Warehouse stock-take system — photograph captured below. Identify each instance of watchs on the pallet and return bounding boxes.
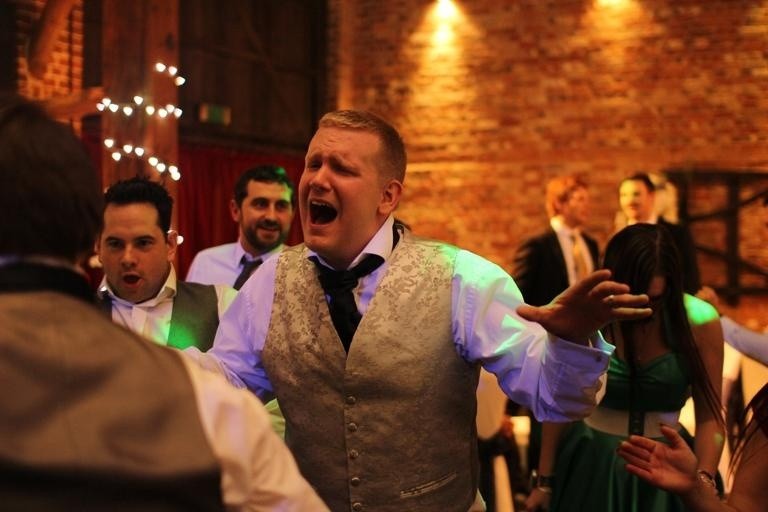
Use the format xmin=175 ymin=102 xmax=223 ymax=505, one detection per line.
xmin=528 ymin=471 xmax=554 ymax=491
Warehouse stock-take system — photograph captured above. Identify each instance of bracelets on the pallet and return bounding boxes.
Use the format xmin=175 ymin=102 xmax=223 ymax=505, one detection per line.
xmin=539 ymin=488 xmax=550 ymax=494
xmin=698 ymin=471 xmax=716 ymax=487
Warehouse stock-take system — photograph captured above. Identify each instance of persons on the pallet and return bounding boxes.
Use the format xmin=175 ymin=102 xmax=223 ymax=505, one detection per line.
xmin=1 ymin=95 xmax=324 ymax=512
xmin=617 ymin=384 xmax=768 ymax=511
xmin=206 ymin=110 xmax=652 ymax=512
xmin=619 ymin=173 xmax=701 ymax=292
xmin=527 ymin=223 xmax=725 ymax=507
xmin=186 ymin=163 xmax=292 ymax=315
xmin=513 ymin=174 xmax=602 ymax=306
xmin=88 ymin=177 xmax=238 ymax=352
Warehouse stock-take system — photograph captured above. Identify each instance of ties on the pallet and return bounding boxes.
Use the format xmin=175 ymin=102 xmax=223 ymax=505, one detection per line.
xmin=231 ymin=254 xmax=264 ymax=291
xmin=302 ymin=220 xmax=400 ymax=358
xmin=569 ymin=233 xmax=591 ymax=283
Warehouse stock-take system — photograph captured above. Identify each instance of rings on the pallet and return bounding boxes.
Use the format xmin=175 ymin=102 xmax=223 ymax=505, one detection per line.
xmin=609 ymin=295 xmax=616 ymax=308
